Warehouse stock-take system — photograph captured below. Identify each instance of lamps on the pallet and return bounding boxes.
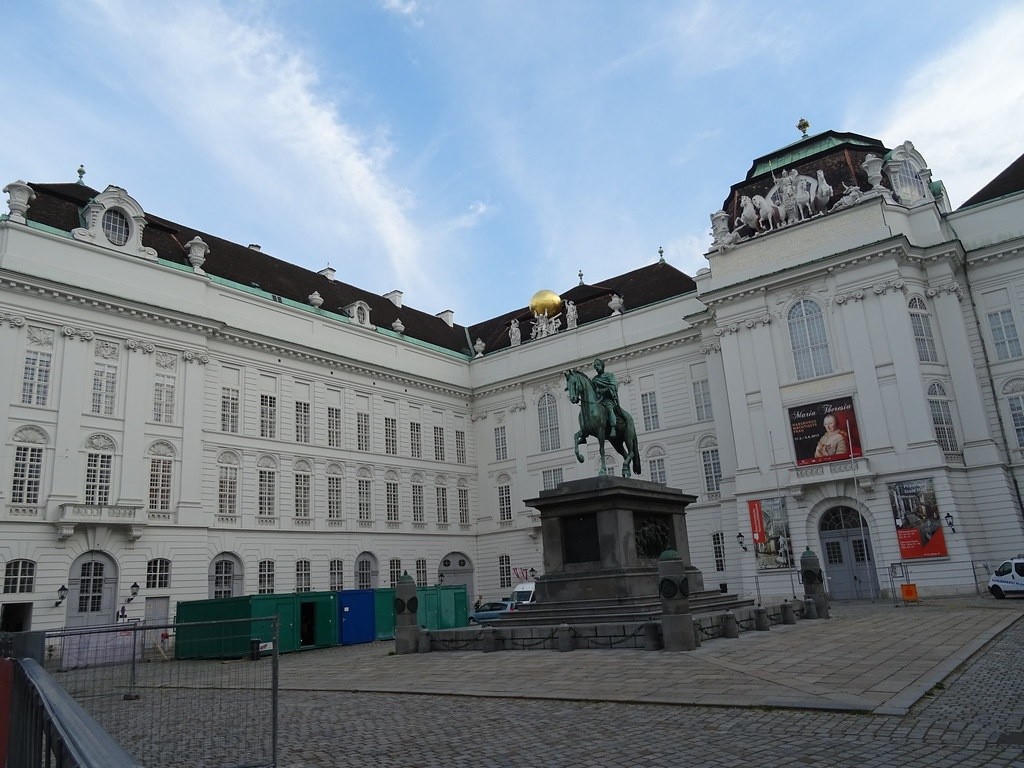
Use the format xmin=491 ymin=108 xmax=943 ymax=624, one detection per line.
xmin=736 ymin=532 xmax=748 ymax=551
xmin=528 ymin=567 xmax=537 ymax=578
xmin=434 ymin=573 xmax=445 ymax=587
xmin=55 ymin=584 xmax=68 ymax=606
xmin=945 ymin=513 xmax=956 ymax=533
xmin=127 ymin=582 xmax=140 ymax=604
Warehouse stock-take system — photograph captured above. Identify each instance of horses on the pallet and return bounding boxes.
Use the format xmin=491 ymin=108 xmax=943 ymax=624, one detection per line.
xmin=564 ymin=368 xmax=645 ymax=479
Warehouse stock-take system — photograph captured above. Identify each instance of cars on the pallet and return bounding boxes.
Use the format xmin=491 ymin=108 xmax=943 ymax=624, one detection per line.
xmin=469 ymin=601 xmax=522 ymax=624
xmin=987 ymin=558 xmax=1024 ymax=599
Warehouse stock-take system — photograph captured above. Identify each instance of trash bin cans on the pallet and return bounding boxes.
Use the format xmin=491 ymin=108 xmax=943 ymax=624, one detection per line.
xmin=900 ymin=583 xmax=918 ymax=600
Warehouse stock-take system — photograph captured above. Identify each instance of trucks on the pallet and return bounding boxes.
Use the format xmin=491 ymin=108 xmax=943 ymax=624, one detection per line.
xmin=508 ymin=582 xmax=536 ymax=604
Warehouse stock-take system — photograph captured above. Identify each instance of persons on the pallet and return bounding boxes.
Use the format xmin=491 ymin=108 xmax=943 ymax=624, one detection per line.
xmin=813 ymin=414 xmax=850 ymax=458
xmin=508 ymin=318 xmax=520 ymax=341
xmin=772 ymin=168 xmax=798 ymax=195
xmin=564 ymin=299 xmax=578 ymax=327
xmin=589 ymin=358 xmax=620 ymax=437
xmin=529 ymin=308 xmax=562 ymax=338
